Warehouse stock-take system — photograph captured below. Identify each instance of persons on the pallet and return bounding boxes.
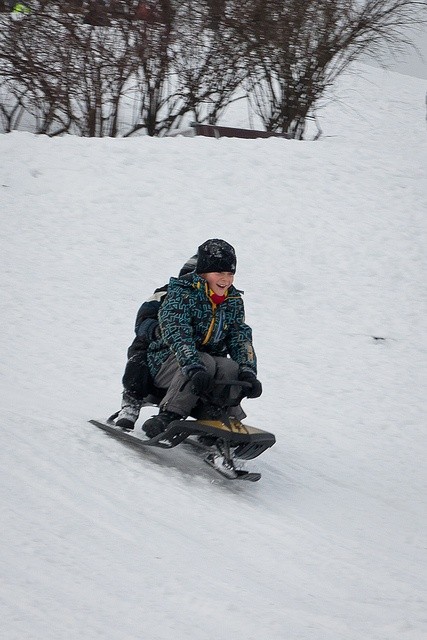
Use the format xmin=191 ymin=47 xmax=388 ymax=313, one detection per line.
xmin=114 ymin=254 xmax=199 ymax=429
xmin=142 ymin=239 xmax=263 ymax=438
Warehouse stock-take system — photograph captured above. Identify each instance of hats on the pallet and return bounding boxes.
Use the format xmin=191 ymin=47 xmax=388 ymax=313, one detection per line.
xmin=196 ymin=239 xmax=236 ymax=274
xmin=180 ymin=254 xmax=199 ymax=276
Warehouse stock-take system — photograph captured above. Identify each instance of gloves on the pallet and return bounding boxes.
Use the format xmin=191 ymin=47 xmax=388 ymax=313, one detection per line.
xmin=239 ymin=371 xmax=262 ymax=398
xmin=187 ymin=370 xmax=210 ymax=394
xmin=155 ymin=325 xmax=162 ymax=339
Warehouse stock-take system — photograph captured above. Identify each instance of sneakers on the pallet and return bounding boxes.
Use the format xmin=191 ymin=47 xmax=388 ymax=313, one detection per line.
xmin=142 ymin=412 xmax=181 ymax=439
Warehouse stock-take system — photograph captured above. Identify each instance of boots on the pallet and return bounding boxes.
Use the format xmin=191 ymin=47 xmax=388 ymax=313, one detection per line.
xmin=115 ymin=392 xmax=142 ymax=428
